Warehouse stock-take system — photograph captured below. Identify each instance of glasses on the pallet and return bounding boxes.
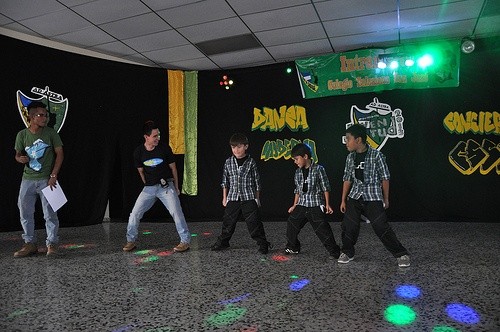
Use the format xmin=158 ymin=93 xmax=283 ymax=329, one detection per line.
xmin=33 ymin=114 xmax=50 ymax=118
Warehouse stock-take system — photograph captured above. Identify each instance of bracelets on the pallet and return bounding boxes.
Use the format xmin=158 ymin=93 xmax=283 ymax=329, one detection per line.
xmin=50 ymin=174 xmax=56 ymax=178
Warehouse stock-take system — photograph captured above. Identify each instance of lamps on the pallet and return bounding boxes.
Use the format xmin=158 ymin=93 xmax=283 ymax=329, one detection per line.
xmin=460 ymin=37 xmax=476 ymax=54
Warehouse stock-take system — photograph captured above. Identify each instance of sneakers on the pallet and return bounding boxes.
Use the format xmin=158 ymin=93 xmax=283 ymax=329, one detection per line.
xmin=258 ymin=244 xmax=267 ymax=254
xmin=286 ymin=246 xmax=301 ymax=254
xmin=14 ymin=244 xmax=36 ymax=258
xmin=336 ymin=253 xmax=354 ymax=263
xmin=46 ymin=245 xmax=56 ymax=258
xmin=175 ymin=241 xmax=190 ymax=253
xmin=123 ymin=241 xmax=137 ymax=251
xmin=397 ymin=253 xmax=412 ymax=267
xmin=329 ymin=250 xmax=339 ymax=260
xmin=210 ymin=242 xmax=231 ymax=250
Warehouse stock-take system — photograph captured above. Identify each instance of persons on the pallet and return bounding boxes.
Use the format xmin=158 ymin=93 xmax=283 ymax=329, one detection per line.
xmin=122 ymin=120 xmax=192 ymax=252
xmin=210 ymin=132 xmax=270 ymax=254
xmin=284 ymin=143 xmax=341 ymax=259
xmin=13 ymin=102 xmax=64 ymax=260
xmin=337 ymin=123 xmax=411 ymax=267
